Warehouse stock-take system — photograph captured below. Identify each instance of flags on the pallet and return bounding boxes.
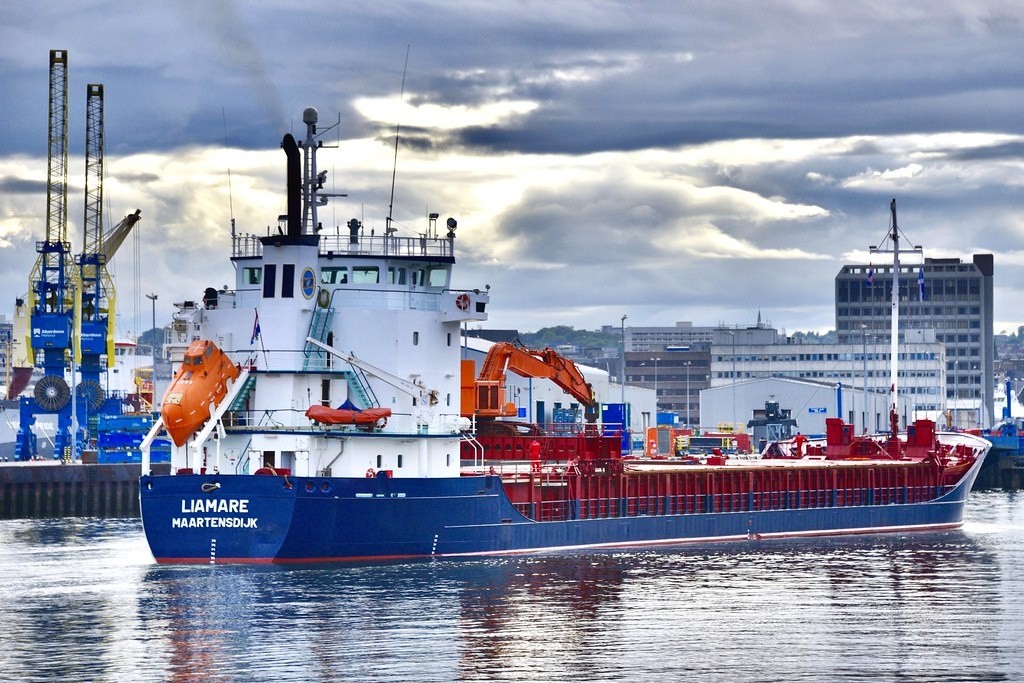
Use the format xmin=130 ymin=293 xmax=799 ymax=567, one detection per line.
xmin=918 ymin=262 xmax=925 ymax=303
xmin=867 ymin=261 xmax=873 ymax=285
xmin=251 ymin=313 xmax=261 ymax=345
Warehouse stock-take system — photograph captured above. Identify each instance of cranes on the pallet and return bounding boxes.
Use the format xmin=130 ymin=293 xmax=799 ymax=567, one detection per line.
xmin=76 ymin=83 xmax=142 ymax=463
xmin=8 ymin=50 xmax=73 ymax=465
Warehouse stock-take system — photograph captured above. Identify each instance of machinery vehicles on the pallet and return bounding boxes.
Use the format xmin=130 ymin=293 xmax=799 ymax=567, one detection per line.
xmin=460 ymin=337 xmax=601 ymax=450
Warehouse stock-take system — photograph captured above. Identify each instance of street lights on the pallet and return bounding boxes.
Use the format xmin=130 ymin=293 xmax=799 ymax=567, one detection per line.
xmin=727 ymin=330 xmax=737 ymax=423
xmin=144 ymin=292 xmax=158 ymax=385
xmin=684 ymin=360 xmax=693 ymax=428
xmin=620 ymin=314 xmax=628 ymax=403
xmin=649 ymin=358 xmax=661 ymax=393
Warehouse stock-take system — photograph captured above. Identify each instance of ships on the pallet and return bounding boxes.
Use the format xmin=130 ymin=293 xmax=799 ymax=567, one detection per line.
xmin=139 ymin=108 xmax=993 ymax=572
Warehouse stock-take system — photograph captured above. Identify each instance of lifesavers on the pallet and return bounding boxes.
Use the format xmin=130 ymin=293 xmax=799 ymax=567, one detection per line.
xmin=366 ymin=468 xmax=376 ymax=478
xmin=456 ymin=295 xmax=469 ymax=310
xmin=319 ymin=290 xmax=330 ymax=308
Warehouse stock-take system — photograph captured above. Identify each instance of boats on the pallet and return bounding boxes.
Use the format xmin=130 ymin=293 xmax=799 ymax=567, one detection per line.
xmin=160 ymin=338 xmax=242 ymax=448
xmin=303 ymin=404 xmax=393 ymax=428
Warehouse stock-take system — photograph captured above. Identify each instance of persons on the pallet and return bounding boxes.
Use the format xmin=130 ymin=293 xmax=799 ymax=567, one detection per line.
xmin=340 ymin=274 xmax=348 ymax=283
xmin=529 ymin=438 xmax=542 ymax=473
xmin=791 ymin=432 xmax=809 ymax=458
